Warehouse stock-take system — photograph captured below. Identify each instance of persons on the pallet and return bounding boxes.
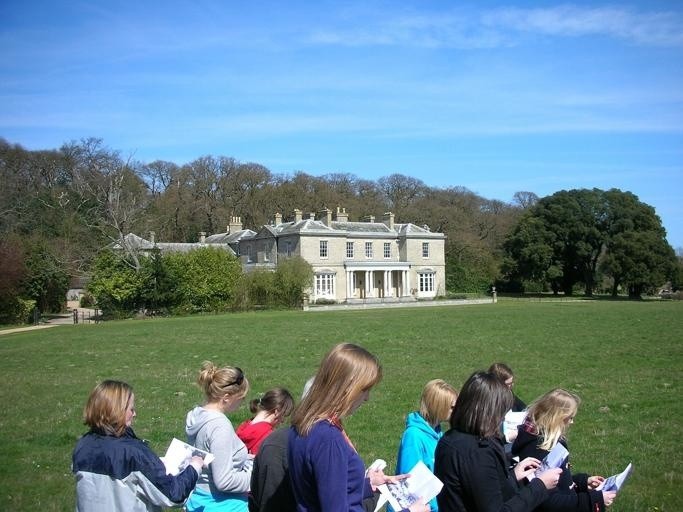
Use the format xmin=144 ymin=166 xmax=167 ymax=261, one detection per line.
xmin=247 ymin=374 xmax=323 ymax=511
xmin=69 ymin=379 xmax=203 ymax=511
xmin=511 ymin=386 xmax=617 ymax=511
xmin=486 ymin=364 xmax=525 ymax=443
xmin=384 ymin=376 xmax=456 ymax=511
xmin=178 ymin=356 xmax=261 ymax=512
xmin=431 ymin=370 xmax=564 ymax=511
xmin=285 ymin=341 xmax=433 ymax=512
xmin=238 ymin=387 xmax=295 ymax=457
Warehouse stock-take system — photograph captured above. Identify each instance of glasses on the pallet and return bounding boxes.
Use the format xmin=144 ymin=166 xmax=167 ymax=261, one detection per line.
xmin=220 ymin=366 xmax=245 ymax=388
